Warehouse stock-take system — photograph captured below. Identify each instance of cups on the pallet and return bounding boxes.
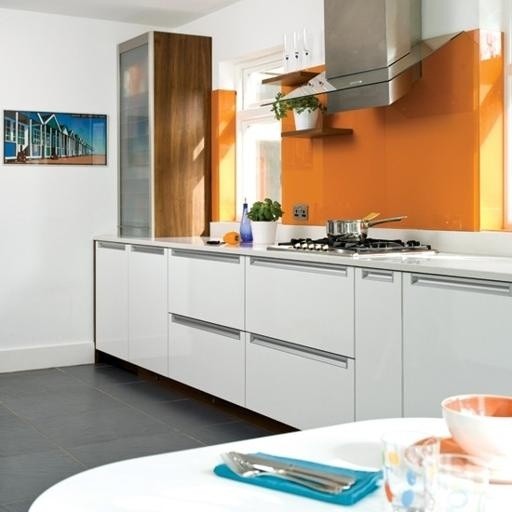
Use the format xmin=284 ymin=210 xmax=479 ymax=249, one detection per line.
xmin=383 ymin=451 xmax=432 ymax=512
xmin=426 ymin=455 xmax=486 ymax=511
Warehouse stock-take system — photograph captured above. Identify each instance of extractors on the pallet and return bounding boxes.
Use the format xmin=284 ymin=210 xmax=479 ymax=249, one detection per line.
xmin=261 ymin=0 xmax=464 ymax=114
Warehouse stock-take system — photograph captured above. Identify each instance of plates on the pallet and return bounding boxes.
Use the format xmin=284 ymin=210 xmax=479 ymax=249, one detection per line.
xmin=404 ymin=431 xmax=512 ymax=483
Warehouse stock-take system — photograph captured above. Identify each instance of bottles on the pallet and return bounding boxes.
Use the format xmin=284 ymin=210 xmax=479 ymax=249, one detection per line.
xmin=223 ymin=232 xmax=239 ymax=245
xmin=280 ymin=28 xmax=315 ymax=73
xmin=239 ymin=199 xmax=252 ymax=243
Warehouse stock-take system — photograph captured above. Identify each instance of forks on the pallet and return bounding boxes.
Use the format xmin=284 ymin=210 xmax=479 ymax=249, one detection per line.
xmin=219 ymin=452 xmax=341 ymax=495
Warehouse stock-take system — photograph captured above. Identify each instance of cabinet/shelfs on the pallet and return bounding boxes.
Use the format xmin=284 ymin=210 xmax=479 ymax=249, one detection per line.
xmin=245 ymin=255 xmax=355 ymax=433
xmin=262 ymin=71 xmax=353 ymax=139
xmin=94 ymin=240 xmax=167 ymax=379
xmin=165 ymin=247 xmax=245 ymax=412
xmin=115 ymin=30 xmax=211 ymax=238
xmin=401 ymin=271 xmax=512 ymax=418
xmin=355 ymin=266 xmax=401 ymax=423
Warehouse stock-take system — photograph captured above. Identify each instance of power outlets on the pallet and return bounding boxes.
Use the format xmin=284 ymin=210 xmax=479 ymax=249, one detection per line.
xmin=292 ymin=206 xmax=308 ymax=220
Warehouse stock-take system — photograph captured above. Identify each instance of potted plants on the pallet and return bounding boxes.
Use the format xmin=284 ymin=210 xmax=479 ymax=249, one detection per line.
xmin=271 ymin=93 xmax=327 ymax=132
xmin=246 ymin=198 xmax=285 ymax=251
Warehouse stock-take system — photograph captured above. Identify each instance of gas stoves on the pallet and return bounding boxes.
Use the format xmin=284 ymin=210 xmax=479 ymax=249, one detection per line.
xmin=266 ymin=236 xmax=441 ymax=258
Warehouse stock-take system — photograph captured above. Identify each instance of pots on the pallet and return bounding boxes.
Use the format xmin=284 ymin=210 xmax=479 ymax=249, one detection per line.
xmin=326 ymin=212 xmax=409 ymax=241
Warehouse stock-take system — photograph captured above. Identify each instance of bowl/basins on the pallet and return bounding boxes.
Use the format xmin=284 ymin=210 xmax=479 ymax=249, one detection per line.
xmin=441 ymin=394 xmax=512 ymax=467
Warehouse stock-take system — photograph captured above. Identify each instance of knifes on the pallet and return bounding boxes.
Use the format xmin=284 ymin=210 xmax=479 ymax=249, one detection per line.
xmin=249 ymin=462 xmax=350 ymax=490
xmin=232 ymin=452 xmax=355 ymax=486
